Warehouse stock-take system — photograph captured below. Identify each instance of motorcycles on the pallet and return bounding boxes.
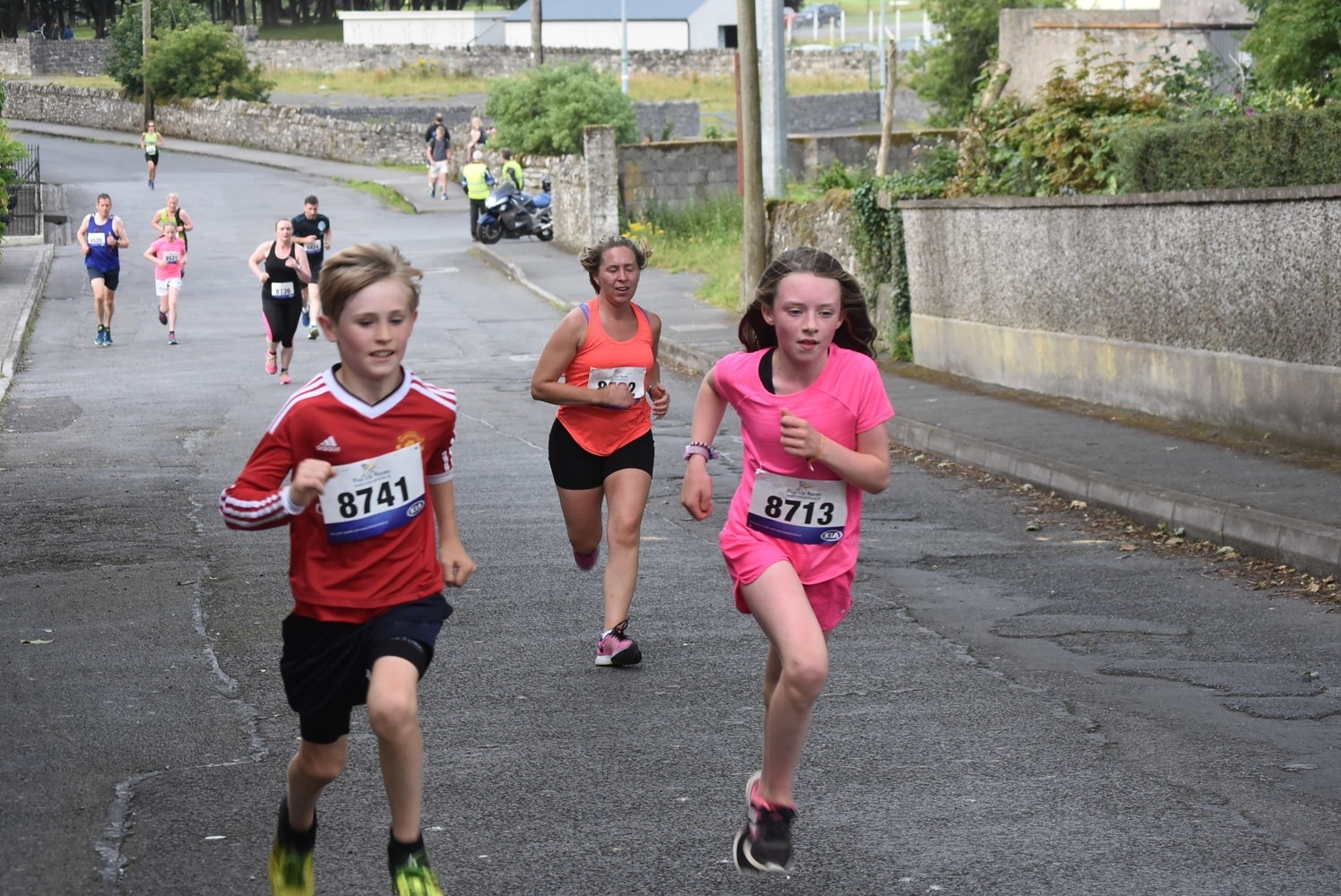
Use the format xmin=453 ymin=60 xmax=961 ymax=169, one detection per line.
xmin=475 ymin=176 xmax=553 ymax=244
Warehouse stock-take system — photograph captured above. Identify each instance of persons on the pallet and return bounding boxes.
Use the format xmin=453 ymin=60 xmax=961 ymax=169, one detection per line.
xmin=680 ymin=246 xmax=895 ymax=880
xmin=151 ymin=193 xmax=194 ymax=278
xmin=530 ymin=236 xmax=671 ymax=666
xmin=218 ymin=244 xmax=477 ymax=896
xmin=423 ymin=110 xmax=524 ymax=243
xmin=77 ymin=193 xmax=129 ymax=347
xmin=143 ymin=221 xmax=187 ymax=346
xmin=247 ymin=217 xmax=312 ymax=385
xmin=140 ymin=120 xmax=165 ymax=190
xmin=290 ymin=194 xmax=332 ymax=340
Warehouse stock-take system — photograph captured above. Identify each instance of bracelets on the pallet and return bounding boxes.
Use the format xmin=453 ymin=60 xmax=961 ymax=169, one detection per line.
xmin=296 ymin=266 xmax=302 ymax=273
xmin=689 ymin=441 xmax=719 ymax=461
xmin=647 ymin=383 xmax=658 ymax=399
xmin=182 ymin=225 xmax=186 ymax=231
xmin=808 ymin=433 xmax=825 ymax=472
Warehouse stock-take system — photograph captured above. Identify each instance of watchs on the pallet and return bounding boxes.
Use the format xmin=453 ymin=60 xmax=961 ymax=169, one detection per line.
xmin=684 ymin=445 xmax=711 ymax=463
xmin=114 ymin=240 xmax=120 ymax=247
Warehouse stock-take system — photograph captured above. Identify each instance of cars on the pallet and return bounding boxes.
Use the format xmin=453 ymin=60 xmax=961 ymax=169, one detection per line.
xmin=784 ymin=4 xmax=841 ymax=28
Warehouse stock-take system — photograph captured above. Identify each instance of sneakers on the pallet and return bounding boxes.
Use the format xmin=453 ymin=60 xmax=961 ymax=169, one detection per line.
xmin=594 ymin=617 xmax=642 ymax=666
xmin=268 ymin=796 xmax=317 ymax=896
xmin=266 ymin=348 xmax=278 ymax=374
xmin=279 ymin=371 xmax=293 ymax=385
xmin=103 ymin=329 xmax=114 ymax=346
xmin=308 ymin=326 xmax=320 ymax=340
xmin=94 ymin=324 xmax=105 ymax=345
xmin=168 ymin=333 xmax=178 ymax=345
xmin=743 ymin=769 xmax=797 ymax=873
xmin=387 ymin=842 xmax=444 ymax=896
xmin=733 ymin=819 xmax=761 ymax=877
xmin=158 ymin=304 xmax=168 ymax=325
xmin=573 ymin=545 xmax=599 ymax=572
xmin=302 ymin=303 xmax=310 ymax=327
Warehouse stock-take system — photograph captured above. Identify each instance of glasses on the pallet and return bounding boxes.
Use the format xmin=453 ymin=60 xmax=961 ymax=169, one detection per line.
xmin=148 ymin=125 xmax=154 ymax=128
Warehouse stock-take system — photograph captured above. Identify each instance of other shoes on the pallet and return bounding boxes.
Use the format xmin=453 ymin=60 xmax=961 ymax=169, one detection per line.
xmin=472 ymin=236 xmax=478 ymax=242
xmin=430 ymin=189 xmax=436 ymax=199
xmin=148 ymin=179 xmax=154 ymax=189
xmin=441 ymin=193 xmax=448 ymax=201
